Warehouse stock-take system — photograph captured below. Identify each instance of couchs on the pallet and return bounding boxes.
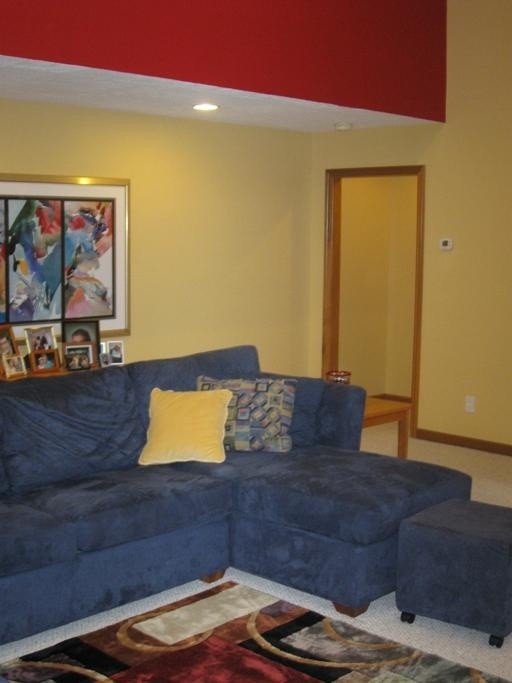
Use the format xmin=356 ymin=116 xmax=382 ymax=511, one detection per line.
xmin=0 ymin=344 xmax=512 ymax=647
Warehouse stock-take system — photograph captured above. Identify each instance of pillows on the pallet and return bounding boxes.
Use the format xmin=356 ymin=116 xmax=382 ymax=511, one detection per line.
xmin=194 ymin=373 xmax=297 ymax=454
xmin=138 ymin=386 xmax=234 ymax=466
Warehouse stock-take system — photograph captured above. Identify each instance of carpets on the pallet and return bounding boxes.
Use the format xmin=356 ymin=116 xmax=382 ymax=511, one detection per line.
xmin=1 ymin=580 xmax=512 ymax=682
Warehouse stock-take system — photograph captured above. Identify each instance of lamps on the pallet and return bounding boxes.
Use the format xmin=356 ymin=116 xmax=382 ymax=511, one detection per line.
xmin=193 ymin=102 xmax=220 ymax=112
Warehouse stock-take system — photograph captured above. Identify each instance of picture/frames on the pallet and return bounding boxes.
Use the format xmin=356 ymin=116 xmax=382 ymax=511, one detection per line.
xmin=1 ymin=352 xmax=26 ymax=378
xmin=23 ymin=323 xmax=59 ymax=377
xmin=65 ymin=344 xmax=93 ymax=370
xmin=61 ymin=320 xmax=104 ymax=373
xmin=0 ymin=324 xmax=22 ymax=380
xmin=67 ymin=354 xmax=90 ymax=371
xmin=0 ymin=170 xmax=132 ymax=339
xmin=29 ymin=347 xmax=62 ymax=375
xmin=105 ymin=340 xmax=124 ymax=365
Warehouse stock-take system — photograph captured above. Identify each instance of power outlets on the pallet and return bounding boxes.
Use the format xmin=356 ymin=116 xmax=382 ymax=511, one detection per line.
xmin=464 ymin=394 xmax=476 ymax=413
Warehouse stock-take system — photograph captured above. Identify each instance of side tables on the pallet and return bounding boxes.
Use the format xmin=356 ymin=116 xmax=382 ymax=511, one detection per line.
xmin=360 ymin=394 xmax=415 ymax=462
